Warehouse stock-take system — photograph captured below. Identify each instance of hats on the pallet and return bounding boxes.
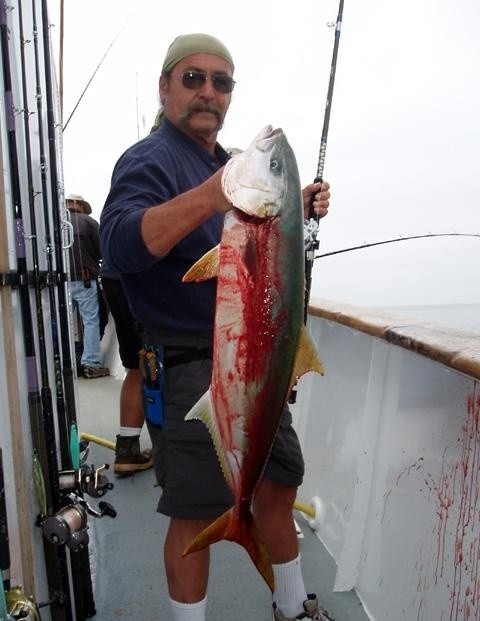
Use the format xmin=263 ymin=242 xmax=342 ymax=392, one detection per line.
xmin=65 ymin=193 xmax=92 ymax=214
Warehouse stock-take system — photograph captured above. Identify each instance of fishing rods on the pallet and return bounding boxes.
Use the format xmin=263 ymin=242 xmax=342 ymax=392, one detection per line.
xmin=314 ymin=234 xmax=478 ymax=259
xmin=1 ymin=0 xmax=97 ymax=620
xmin=63 ymin=26 xmax=140 ymax=142
xmin=288 ymin=0 xmax=343 ymax=403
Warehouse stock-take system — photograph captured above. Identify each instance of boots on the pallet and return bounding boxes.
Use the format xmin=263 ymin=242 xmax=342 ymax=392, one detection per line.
xmin=113 ymin=434 xmax=154 ymax=474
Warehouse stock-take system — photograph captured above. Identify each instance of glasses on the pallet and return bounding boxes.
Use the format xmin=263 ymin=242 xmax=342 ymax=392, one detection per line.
xmin=179 ymin=70 xmax=236 ymax=94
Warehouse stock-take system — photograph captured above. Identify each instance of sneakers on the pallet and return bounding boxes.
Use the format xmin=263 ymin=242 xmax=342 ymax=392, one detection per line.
xmin=272 ymin=594 xmax=339 ymax=621
xmin=82 ymin=363 xmax=109 ymax=379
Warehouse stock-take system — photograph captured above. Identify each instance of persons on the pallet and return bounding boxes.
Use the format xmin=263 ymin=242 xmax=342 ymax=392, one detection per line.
xmin=65 ymin=194 xmax=110 ymax=379
xmin=97 ymin=28 xmax=341 ymax=621
xmin=99 ymin=254 xmax=155 ymax=475
